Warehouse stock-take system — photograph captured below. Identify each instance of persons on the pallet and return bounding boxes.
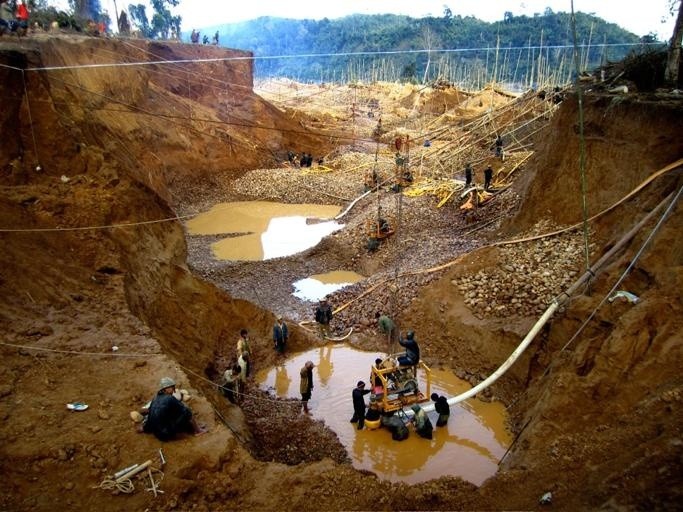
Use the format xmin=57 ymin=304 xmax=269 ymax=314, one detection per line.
xmin=484 ymin=165 xmax=493 ymax=189
xmin=0 ymin=0 xmax=30 ymax=38
xmin=288 ymin=151 xmax=312 ymax=167
xmin=315 ymin=298 xmax=333 ymax=338
xmin=465 ymin=163 xmax=473 ymax=186
xmin=135 ymin=377 xmax=209 ymax=441
xmin=221 ymin=329 xmax=252 ymax=403
xmin=398 ymin=330 xmax=419 ymax=366
xmin=350 ymin=358 xmax=450 ymax=440
xmin=496 ymin=136 xmax=502 ymax=157
xmin=375 ymin=312 xmax=398 ymax=344
xmin=300 ymin=360 xmax=314 ymax=414
xmin=273 ymin=315 xmax=289 ymax=358
xmin=191 ymin=28 xmax=220 ymax=45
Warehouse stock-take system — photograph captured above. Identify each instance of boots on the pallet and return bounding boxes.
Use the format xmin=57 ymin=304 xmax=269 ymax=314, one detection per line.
xmin=303 ymin=402 xmax=312 ymax=412
xmin=278 ymin=351 xmax=288 ymax=358
xmin=190 ymin=416 xmax=208 ymax=436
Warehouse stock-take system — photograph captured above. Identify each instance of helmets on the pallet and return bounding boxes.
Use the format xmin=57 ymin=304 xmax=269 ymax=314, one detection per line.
xmin=277 ymin=315 xmax=283 ymax=320
xmin=406 ymin=331 xmax=414 ymax=336
xmin=357 ymin=381 xmax=365 ymax=388
xmin=159 ymin=377 xmax=176 ymax=391
xmin=319 ymin=297 xmax=327 ymax=302
xmin=488 ymin=165 xmax=492 ymax=167
